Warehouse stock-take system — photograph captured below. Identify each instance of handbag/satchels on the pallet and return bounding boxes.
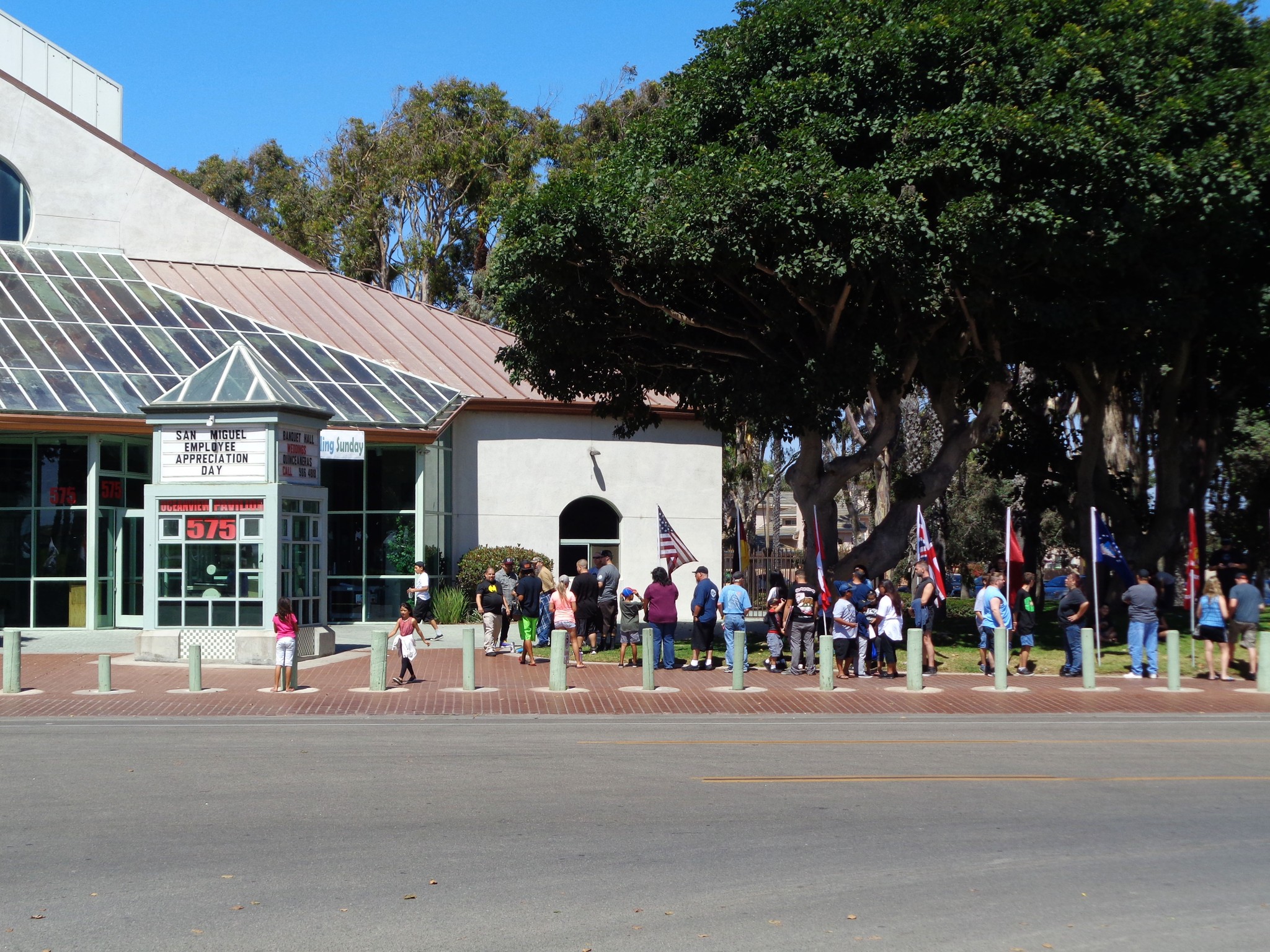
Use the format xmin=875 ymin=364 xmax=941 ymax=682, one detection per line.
xmin=512 ymin=596 xmax=522 ymax=620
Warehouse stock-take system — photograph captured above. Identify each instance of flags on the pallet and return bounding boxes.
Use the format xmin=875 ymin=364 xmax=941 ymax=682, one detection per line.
xmin=813 ymin=509 xmax=834 ymax=619
xmin=916 ymin=505 xmax=947 ymax=609
xmin=1006 ymin=507 xmax=1026 ymax=611
xmin=1090 ymin=507 xmax=1136 ymax=588
xmin=656 ymin=505 xmax=699 ymax=581
xmin=731 ymin=504 xmax=750 ymax=575
xmin=1182 ymin=511 xmax=1200 ymax=611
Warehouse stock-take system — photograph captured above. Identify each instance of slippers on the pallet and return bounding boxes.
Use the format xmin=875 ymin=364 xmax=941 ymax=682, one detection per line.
xmin=528 ymin=661 xmax=537 ymax=665
xmin=270 ymin=689 xmax=277 ymax=692
xmin=518 ymin=656 xmax=525 ymax=664
xmin=288 ymin=687 xmax=295 ymax=692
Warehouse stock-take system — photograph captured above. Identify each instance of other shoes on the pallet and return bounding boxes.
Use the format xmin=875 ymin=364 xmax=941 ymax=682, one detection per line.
xmin=393 ymin=678 xmax=402 ymax=685
xmin=570 ymin=640 xmax=639 ymax=668
xmin=534 ymin=642 xmax=549 ymax=649
xmin=407 ymin=676 xmax=416 ymax=682
xmin=486 ymin=652 xmax=496 ymax=656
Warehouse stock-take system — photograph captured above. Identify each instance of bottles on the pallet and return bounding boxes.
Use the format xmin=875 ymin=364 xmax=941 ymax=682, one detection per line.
xmin=516 ymin=648 xmax=523 ymax=653
xmin=408 ymin=588 xmax=413 ymax=599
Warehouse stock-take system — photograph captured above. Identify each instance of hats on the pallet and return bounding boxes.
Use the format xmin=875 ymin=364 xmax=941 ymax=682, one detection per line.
xmin=1235 ymin=573 xmax=1248 ymax=579
xmin=692 ymin=566 xmax=708 ymax=574
xmin=622 ymin=587 xmax=636 ymax=597
xmin=1139 ymin=569 xmax=1149 ymax=578
xmin=598 ymin=550 xmax=613 ymax=557
xmin=839 ymin=584 xmax=856 ymax=593
xmin=858 ymin=600 xmax=871 ymax=607
xmin=415 ymin=561 xmax=426 ymax=568
xmin=732 ymin=571 xmax=747 ymax=579
xmin=503 ymin=557 xmax=543 ymax=571
xmin=559 ymin=575 xmax=570 ymax=584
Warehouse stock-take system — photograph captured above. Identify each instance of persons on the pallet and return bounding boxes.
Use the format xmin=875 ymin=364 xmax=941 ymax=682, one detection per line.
xmin=643 ymin=567 xmax=679 ymax=670
xmin=388 ymin=602 xmax=430 ymax=685
xmin=681 ymin=566 xmax=719 ymax=671
xmin=476 ymin=557 xmax=558 ymax=666
xmin=270 ymin=599 xmax=299 ymax=692
xmin=909 ymin=560 xmax=937 ymax=676
xmin=1196 ymin=535 xmax=1265 ymax=682
xmin=973 ymin=556 xmax=1036 ymax=677
xmin=717 ymin=571 xmax=752 ymax=673
xmin=225 ymin=558 xmax=249 ymax=598
xmin=617 ymin=587 xmax=644 ymax=668
xmin=762 ymin=565 xmax=906 ymax=679
xmin=1043 ymin=549 xmax=1175 ymax=679
xmin=407 ymin=562 xmax=444 ymax=641
xmin=570 ymin=549 xmax=620 ymax=655
xmin=548 ymin=575 xmax=587 ymax=669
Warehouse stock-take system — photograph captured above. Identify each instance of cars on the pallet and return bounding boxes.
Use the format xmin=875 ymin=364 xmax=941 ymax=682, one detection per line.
xmin=754 ymin=573 xmax=792 ymax=592
xmin=944 ymin=573 xmax=984 ymax=598
xmin=1041 ymin=575 xmax=1087 ymax=602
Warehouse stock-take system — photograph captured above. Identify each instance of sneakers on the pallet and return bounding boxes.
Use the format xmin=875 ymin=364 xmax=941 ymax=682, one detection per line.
xmin=654 ymin=659 xmax=936 ymax=678
xmin=430 ymin=634 xmax=443 ymax=641
xmin=980 ymin=665 xmax=1255 ymax=681
xmin=499 ymin=641 xmax=511 ymax=647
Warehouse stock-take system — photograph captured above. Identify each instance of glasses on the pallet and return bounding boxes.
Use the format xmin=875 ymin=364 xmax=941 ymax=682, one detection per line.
xmin=867 ymin=598 xmax=876 ymax=602
xmin=593 ymin=556 xmax=600 ymax=559
xmin=879 ymin=586 xmax=884 ymax=588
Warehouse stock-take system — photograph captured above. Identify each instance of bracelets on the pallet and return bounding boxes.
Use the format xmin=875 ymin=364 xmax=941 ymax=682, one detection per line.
xmin=514 ymin=593 xmax=517 ymax=594
xmin=721 ymin=615 xmax=724 ymax=617
xmin=638 ymin=596 xmax=641 ymax=599
xmin=693 ymin=615 xmax=697 ymax=618
